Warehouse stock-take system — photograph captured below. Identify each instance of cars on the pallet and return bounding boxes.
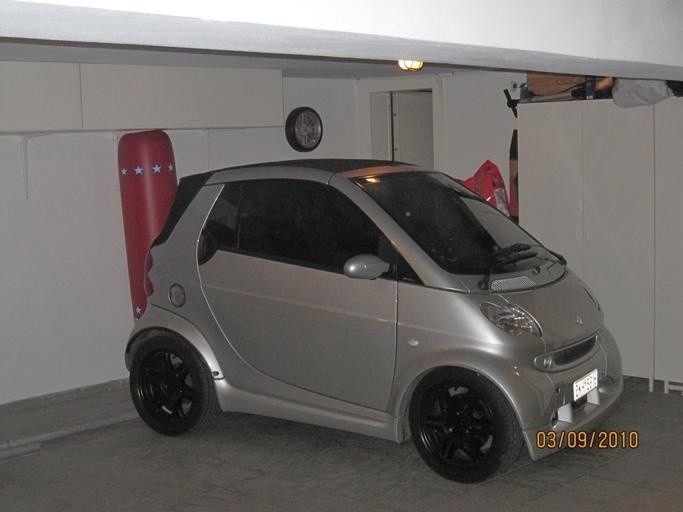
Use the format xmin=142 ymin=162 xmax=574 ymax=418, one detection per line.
xmin=124 ymin=158 xmax=624 ymax=484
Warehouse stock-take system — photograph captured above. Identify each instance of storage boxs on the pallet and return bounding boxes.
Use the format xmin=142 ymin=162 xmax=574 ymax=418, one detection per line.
xmin=613 ymin=79 xmax=672 ymax=108
xmin=527 ymin=77 xmax=590 ymax=93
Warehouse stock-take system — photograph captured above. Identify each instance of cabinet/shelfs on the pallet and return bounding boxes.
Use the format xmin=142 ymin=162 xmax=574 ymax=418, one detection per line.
xmin=520 ymin=100 xmax=655 ymax=391
xmin=653 ymin=100 xmax=682 ymax=393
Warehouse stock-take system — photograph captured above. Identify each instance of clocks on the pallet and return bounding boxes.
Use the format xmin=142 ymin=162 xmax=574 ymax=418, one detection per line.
xmin=285 ymin=107 xmax=324 ymax=152
